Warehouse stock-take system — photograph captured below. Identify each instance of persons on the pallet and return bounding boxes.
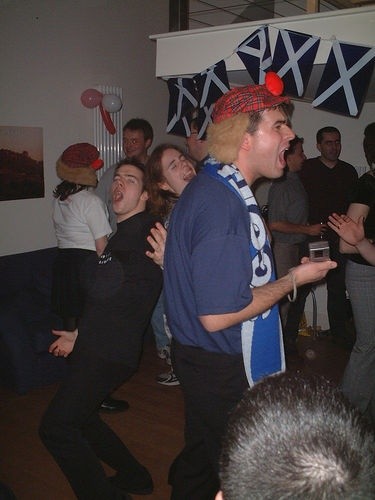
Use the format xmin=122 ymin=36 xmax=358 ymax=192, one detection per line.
xmin=38 ymin=155 xmax=163 ymax=500
xmin=163 ymin=71 xmax=338 ymax=500
xmin=267 ymin=122 xmax=375 ymax=425
xmin=51 ymin=106 xmax=211 ymax=410
xmin=216 ymin=370 xmax=375 ymax=500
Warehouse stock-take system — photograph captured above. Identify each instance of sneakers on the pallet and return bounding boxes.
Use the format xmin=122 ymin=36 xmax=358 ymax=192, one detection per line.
xmin=155 ymin=370 xmax=180 ymax=386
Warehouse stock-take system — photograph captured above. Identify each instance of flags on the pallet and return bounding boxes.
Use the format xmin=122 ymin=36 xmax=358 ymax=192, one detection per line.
xmin=166 ymin=59 xmax=231 ymax=140
xmin=272 ymin=28 xmax=320 ymax=99
xmin=233 ymin=25 xmax=271 ymax=86
xmin=312 ymin=40 xmax=375 ymax=119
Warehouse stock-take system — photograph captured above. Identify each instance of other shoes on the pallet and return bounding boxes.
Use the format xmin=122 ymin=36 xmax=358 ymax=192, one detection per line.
xmin=101 ymin=398 xmax=129 ymax=411
xmin=109 ymin=473 xmax=153 ymax=495
xmin=331 ymin=336 xmax=355 ymax=351
xmin=286 ymin=345 xmax=299 ymax=370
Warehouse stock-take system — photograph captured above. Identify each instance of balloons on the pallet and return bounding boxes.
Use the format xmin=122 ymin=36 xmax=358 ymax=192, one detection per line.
xmin=81 ymin=89 xmax=121 ymax=134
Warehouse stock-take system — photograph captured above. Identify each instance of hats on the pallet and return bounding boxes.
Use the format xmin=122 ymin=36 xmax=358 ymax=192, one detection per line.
xmin=56 ymin=142 xmax=103 ymax=187
xmin=210 ymin=71 xmax=291 ymax=123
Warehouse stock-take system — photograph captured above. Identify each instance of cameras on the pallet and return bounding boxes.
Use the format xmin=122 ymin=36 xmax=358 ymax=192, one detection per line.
xmin=308 ymin=241 xmax=331 ymax=262
